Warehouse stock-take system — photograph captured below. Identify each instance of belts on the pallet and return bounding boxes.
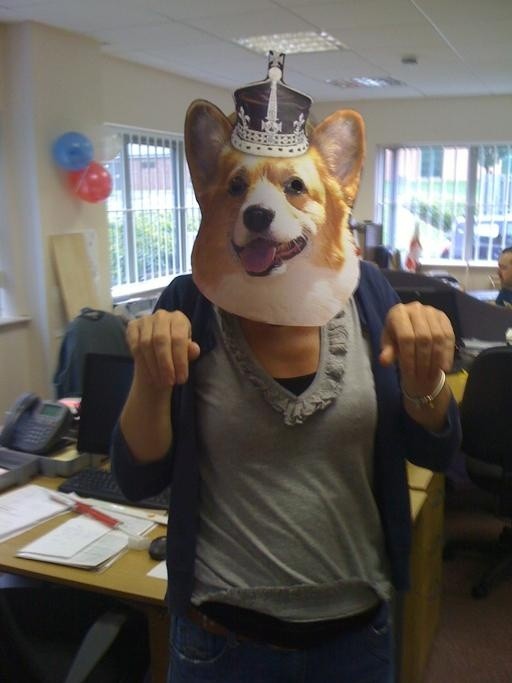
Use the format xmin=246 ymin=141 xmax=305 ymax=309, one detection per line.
xmin=181 ymin=602 xmax=297 ymax=653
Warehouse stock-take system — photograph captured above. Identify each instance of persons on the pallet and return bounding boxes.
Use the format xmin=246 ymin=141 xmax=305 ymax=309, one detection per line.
xmin=111 ymin=43 xmax=468 ymax=681
xmin=493 ymin=245 xmax=512 ymax=307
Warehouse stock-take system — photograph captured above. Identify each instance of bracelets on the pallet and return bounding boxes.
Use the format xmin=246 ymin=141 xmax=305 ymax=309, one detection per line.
xmin=399 ymin=369 xmax=446 ymax=411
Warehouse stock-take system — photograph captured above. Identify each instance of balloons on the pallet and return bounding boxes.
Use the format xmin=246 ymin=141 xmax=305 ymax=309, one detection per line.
xmin=69 ymin=160 xmax=114 ymax=202
xmin=50 ymin=127 xmax=96 ymax=171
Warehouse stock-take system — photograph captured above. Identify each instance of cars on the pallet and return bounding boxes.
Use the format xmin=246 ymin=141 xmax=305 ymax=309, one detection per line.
xmin=453 ymin=214 xmax=512 ymax=261
xmin=378 ymin=199 xmax=454 ymax=262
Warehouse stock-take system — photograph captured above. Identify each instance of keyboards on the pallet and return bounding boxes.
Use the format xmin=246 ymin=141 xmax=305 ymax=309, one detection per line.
xmin=58 ymin=466 xmax=168 ymax=510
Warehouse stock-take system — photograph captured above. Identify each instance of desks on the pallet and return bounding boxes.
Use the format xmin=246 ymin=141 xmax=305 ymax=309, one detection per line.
xmin=406 ymin=459 xmax=445 ymax=683
xmin=0 ymin=475 xmax=428 ymax=683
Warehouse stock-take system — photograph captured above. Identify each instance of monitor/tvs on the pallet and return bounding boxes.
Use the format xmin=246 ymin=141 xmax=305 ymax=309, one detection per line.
xmin=77 ymin=353 xmax=134 ymax=455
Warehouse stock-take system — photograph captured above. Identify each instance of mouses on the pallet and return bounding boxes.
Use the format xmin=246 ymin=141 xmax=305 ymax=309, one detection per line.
xmin=149 ymin=536 xmax=167 ymax=560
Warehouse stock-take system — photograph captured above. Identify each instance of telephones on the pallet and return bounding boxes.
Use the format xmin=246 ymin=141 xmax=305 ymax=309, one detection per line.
xmin=0 ymin=392 xmax=73 ymax=454
